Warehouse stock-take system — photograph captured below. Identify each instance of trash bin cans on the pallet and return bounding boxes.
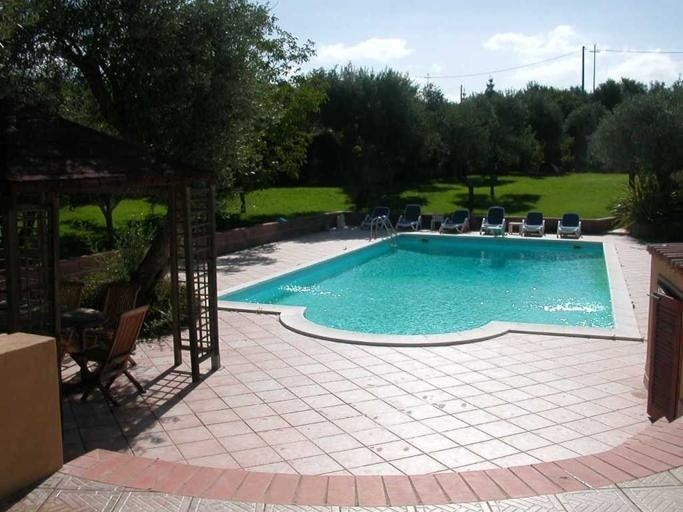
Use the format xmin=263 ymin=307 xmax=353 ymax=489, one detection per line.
xmin=337 ymin=214 xmax=344 ymax=228
xmin=644 ymin=242 xmax=683 ymax=417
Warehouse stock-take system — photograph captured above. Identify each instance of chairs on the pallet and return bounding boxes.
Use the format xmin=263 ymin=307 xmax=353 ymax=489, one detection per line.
xmin=359 ymin=204 xmax=582 ymax=240
xmin=50 ymin=283 xmax=151 ymax=451
